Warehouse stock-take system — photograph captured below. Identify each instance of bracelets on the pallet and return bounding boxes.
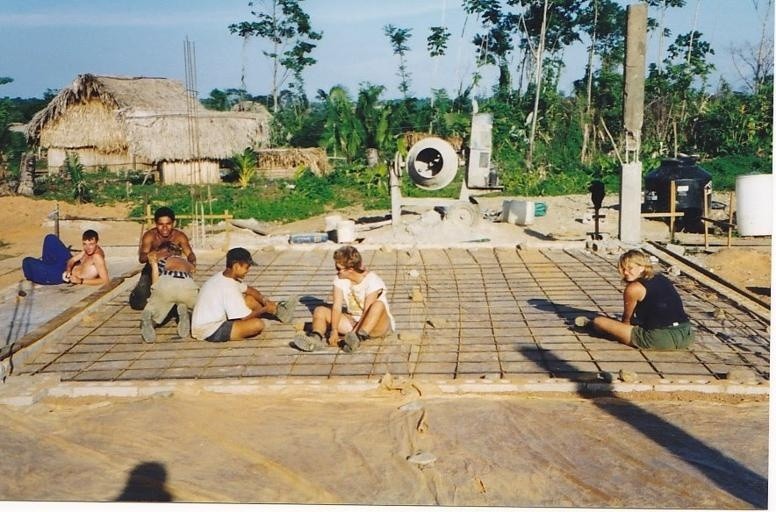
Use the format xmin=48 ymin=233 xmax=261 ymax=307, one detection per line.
xmin=80 ymin=278 xmax=84 ymax=284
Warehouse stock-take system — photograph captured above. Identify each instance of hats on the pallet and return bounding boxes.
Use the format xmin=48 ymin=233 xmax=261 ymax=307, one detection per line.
xmin=226 ymin=248 xmax=258 ymax=266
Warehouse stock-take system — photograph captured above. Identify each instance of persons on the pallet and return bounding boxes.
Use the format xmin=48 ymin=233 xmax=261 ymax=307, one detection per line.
xmin=572 ymin=250 xmax=693 ymax=351
xmin=293 ymin=245 xmax=394 ymax=352
xmin=141 ymin=242 xmax=196 ymax=343
xmin=189 ymin=247 xmax=295 ymax=343
xmin=128 ymin=206 xmax=196 ymax=310
xmin=22 ymin=229 xmax=108 ymax=285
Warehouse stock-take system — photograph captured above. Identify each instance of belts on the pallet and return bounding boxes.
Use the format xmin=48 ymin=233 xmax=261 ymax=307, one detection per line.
xmin=173 ymin=276 xmax=184 ymax=279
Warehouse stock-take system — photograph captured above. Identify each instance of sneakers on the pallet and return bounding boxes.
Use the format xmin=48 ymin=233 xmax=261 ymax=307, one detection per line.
xmin=176 ymin=303 xmax=191 ymax=337
xmin=141 ymin=309 xmax=156 ymax=343
xmin=343 ymin=332 xmax=360 ymax=352
xmin=293 ymin=333 xmax=325 ymax=351
xmin=335 ymin=266 xmax=347 ymax=271
xmin=574 ymin=316 xmax=591 ymax=328
xmin=275 ymin=294 xmax=297 ymax=324
